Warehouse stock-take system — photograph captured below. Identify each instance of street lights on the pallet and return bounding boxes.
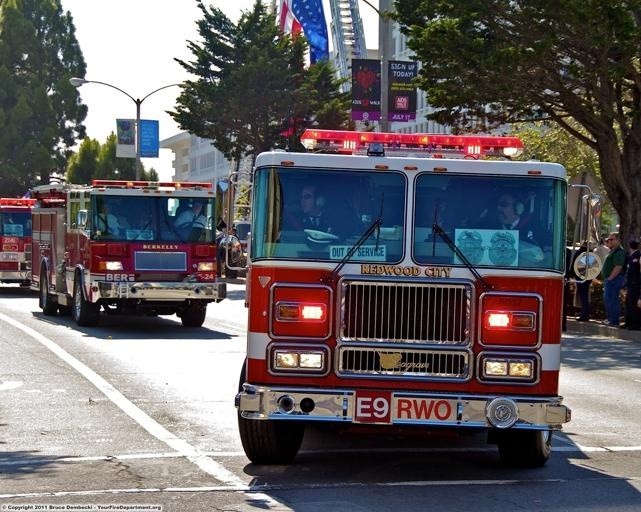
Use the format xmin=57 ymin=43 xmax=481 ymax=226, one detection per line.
xmin=69 ymin=77 xmax=188 ymax=181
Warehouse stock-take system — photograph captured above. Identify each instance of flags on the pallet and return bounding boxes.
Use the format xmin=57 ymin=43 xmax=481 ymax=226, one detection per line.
xmin=277 ymin=0 xmax=330 ymax=141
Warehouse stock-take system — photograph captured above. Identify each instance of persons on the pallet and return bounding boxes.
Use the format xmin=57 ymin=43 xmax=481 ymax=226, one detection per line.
xmin=574 ymin=239 xmax=595 ymax=323
xmin=620 ymin=237 xmax=641 ymax=331
xmin=593 ymin=237 xmax=612 ymax=259
xmin=175 ymin=198 xmax=207 ymax=229
xmin=95 ymin=203 xmax=121 ymax=237
xmin=288 ymin=180 xmax=342 ymax=238
xmin=215 ymin=220 xmax=241 ymax=279
xmin=494 ymin=193 xmax=540 ymax=248
xmin=601 ymin=233 xmax=626 ymax=326
xmin=113 ymin=204 xmax=131 ymax=228
xmin=562 ymin=247 xmax=573 ymax=333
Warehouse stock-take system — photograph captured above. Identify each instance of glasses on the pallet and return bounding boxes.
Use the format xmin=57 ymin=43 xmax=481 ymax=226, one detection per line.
xmin=609 ymin=238 xmax=613 ymax=240
xmin=495 ymin=200 xmax=516 ymax=207
xmin=300 ymin=194 xmax=317 ymax=200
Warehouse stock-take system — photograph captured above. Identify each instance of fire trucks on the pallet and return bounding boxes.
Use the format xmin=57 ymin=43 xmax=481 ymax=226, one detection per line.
xmin=234 ymin=128 xmax=601 ymax=466
xmin=30 ymin=179 xmax=227 ymax=329
xmin=0 ymin=197 xmax=36 ymax=285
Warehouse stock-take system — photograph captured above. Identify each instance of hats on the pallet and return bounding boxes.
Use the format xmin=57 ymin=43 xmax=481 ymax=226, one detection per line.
xmin=304 ymin=229 xmax=339 ymax=244
